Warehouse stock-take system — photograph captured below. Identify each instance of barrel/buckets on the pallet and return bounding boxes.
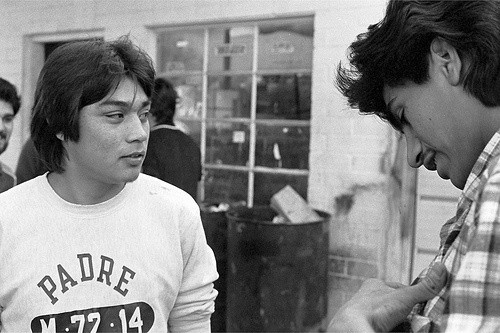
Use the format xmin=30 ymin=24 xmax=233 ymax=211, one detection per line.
xmin=226 ymin=205 xmax=332 ymax=332
xmin=198 ymin=201 xmax=248 ymax=332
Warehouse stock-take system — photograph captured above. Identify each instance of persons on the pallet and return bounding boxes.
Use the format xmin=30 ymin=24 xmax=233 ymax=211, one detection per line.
xmin=325 ymin=0 xmax=500 ymax=333
xmin=0 ymin=33 xmax=220 ymax=333
xmin=0 ymin=77 xmax=21 ymax=193
xmin=140 ymin=76 xmax=204 ymax=216
xmin=15 ymin=134 xmax=50 ymax=186
